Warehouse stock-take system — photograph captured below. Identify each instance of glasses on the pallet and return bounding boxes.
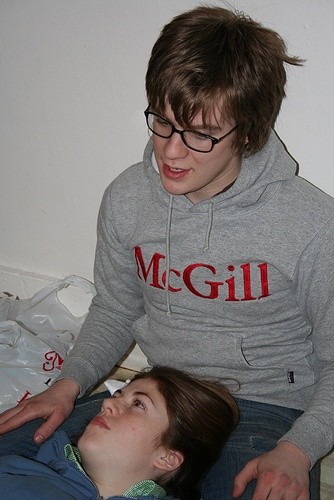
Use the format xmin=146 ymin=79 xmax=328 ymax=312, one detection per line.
xmin=144 ymin=103 xmax=240 ymax=153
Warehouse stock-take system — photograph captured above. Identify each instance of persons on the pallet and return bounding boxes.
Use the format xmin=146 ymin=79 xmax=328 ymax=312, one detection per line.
xmin=0 ymin=367 xmax=240 ymax=500
xmin=0 ymin=7 xmax=334 ymax=500
